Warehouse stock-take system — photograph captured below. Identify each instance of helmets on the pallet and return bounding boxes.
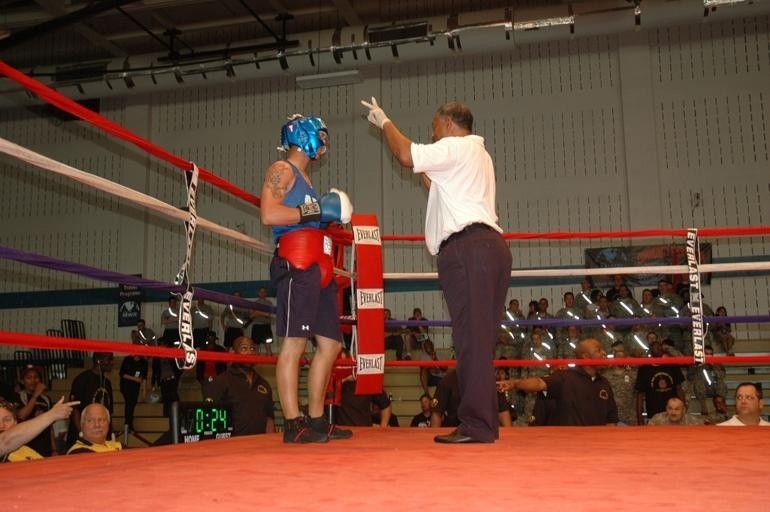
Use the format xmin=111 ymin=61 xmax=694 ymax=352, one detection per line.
xmin=277 ymin=114 xmax=328 ymax=160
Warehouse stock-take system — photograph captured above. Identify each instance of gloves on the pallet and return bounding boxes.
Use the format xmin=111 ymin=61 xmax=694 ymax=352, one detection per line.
xmin=319 ymin=188 xmax=353 ymax=224
xmin=361 ymin=97 xmax=391 ymax=130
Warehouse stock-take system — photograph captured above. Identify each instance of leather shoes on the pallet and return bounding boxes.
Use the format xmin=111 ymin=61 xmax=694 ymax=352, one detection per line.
xmin=434 ymin=430 xmax=477 ymax=442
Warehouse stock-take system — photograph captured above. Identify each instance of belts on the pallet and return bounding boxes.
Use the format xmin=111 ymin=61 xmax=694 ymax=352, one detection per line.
xmin=440 ymin=224 xmax=493 ymax=245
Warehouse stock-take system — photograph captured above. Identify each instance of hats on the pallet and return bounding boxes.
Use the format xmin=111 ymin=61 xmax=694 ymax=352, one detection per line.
xmin=20 ymin=364 xmax=44 ymax=379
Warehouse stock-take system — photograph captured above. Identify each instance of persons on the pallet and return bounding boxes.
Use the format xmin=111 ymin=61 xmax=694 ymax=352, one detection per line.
xmin=497 ymin=275 xmax=735 ymax=425
xmin=370 ymin=399 xmax=400 ymax=428
xmin=382 ymin=307 xmax=403 ymax=361
xmin=430 ymin=361 xmax=512 ymax=425
xmin=360 ymin=95 xmax=516 ymax=445
xmin=120 ymin=286 xmax=275 ymax=435
xmin=404 ymin=308 xmax=430 ymax=359
xmin=316 ymin=340 xmax=393 ymax=428
xmin=1 ymin=363 xmax=130 ymax=464
xmin=260 ymin=109 xmax=357 ymax=442
xmin=203 ymin=335 xmax=275 ymax=438
xmin=411 ymin=394 xmax=432 ymax=426
xmin=419 ymin=338 xmax=448 ymax=394
xmin=719 ymin=382 xmax=770 ymax=425
xmin=66 ymin=351 xmax=114 ymax=444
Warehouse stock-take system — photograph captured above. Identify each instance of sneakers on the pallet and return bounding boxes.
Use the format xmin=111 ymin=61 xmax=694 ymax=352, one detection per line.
xmin=283 ymin=415 xmax=352 ymax=443
